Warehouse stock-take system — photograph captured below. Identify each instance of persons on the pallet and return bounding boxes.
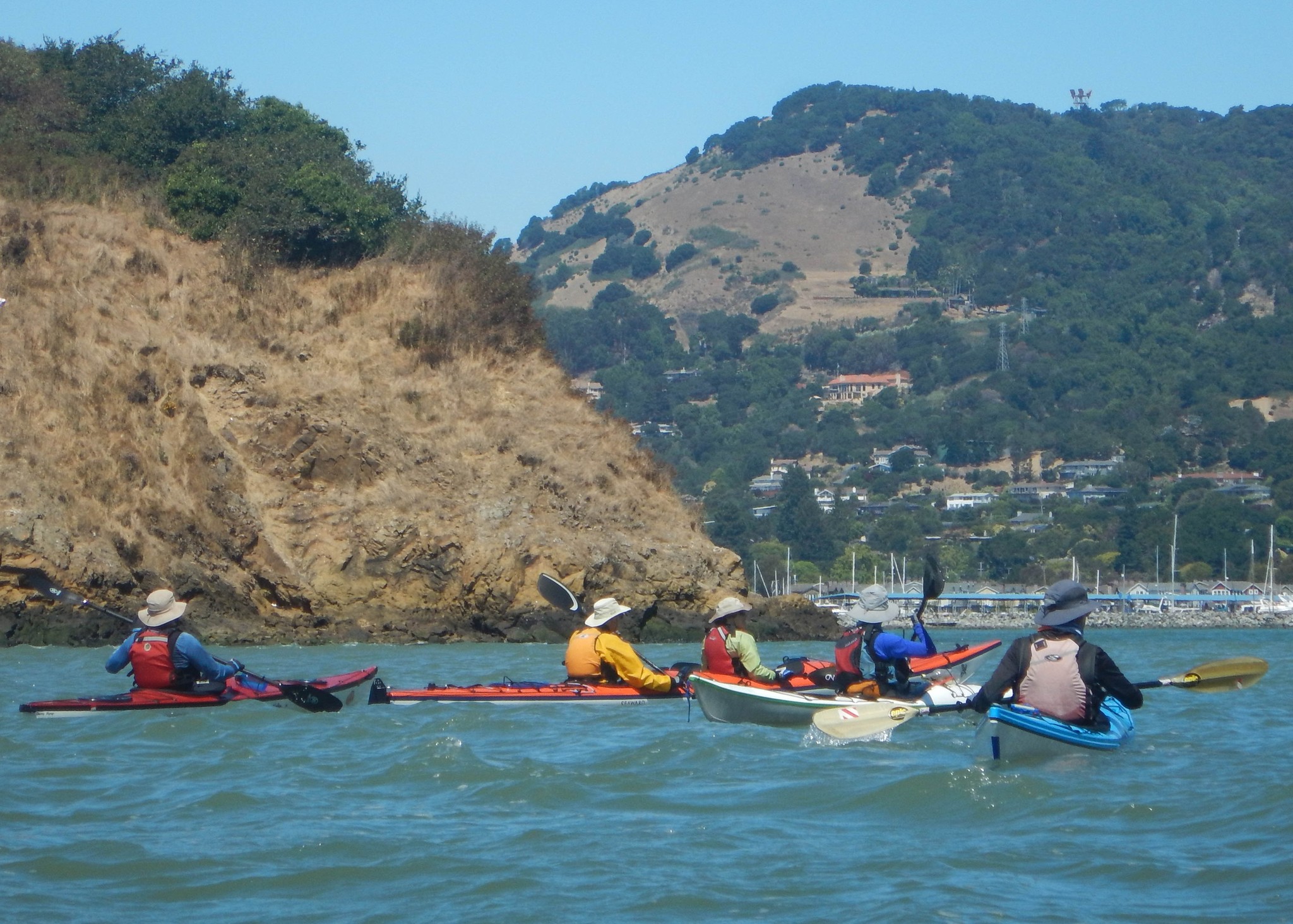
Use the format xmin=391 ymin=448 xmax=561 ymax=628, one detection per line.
xmin=700 ymin=595 xmax=795 ymax=684
xmin=846 ymin=584 xmax=938 ymax=700
xmin=564 ymin=598 xmax=683 ymax=694
xmin=966 ymin=579 xmax=1144 ymax=733
xmin=104 ymin=590 xmax=245 ymax=693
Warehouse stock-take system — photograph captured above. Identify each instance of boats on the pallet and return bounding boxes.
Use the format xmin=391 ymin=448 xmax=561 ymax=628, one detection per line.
xmin=968 ymin=694 xmax=1138 ymax=758
xmin=368 ymin=657 xmax=838 ymax=707
xmin=19 ymin=662 xmax=381 ymax=718
xmin=682 ymin=667 xmax=1019 ymax=730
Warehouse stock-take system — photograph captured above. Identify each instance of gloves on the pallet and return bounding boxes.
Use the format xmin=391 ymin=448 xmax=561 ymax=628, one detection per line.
xmin=911 ymin=613 xmax=924 ymax=624
xmin=779 ymin=669 xmax=794 ymax=683
xmin=674 ymin=666 xmax=693 ymax=685
xmin=227 ymin=659 xmax=246 ymax=672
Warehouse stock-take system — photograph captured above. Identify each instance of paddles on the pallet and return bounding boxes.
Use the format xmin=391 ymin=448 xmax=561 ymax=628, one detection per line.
xmin=911 ymin=554 xmax=945 ymax=642
xmin=813 ymin=657 xmax=1271 ymax=739
xmin=536 ymin=572 xmax=697 ymax=699
xmin=671 ymin=662 xmax=839 ymax=690
xmin=26 ymin=567 xmax=343 ymax=713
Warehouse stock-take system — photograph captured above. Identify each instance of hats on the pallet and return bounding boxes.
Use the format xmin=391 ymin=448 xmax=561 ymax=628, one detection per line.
xmin=138 ymin=589 xmax=187 ymax=627
xmin=846 ymin=584 xmax=899 ymax=623
xmin=709 ymin=598 xmax=752 ymax=624
xmin=585 ymin=598 xmax=631 ymax=627
xmin=1034 ymin=579 xmax=1098 ymax=625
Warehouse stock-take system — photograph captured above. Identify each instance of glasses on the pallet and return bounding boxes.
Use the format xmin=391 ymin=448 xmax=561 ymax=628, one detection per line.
xmin=736 ymin=611 xmax=747 ymax=616
